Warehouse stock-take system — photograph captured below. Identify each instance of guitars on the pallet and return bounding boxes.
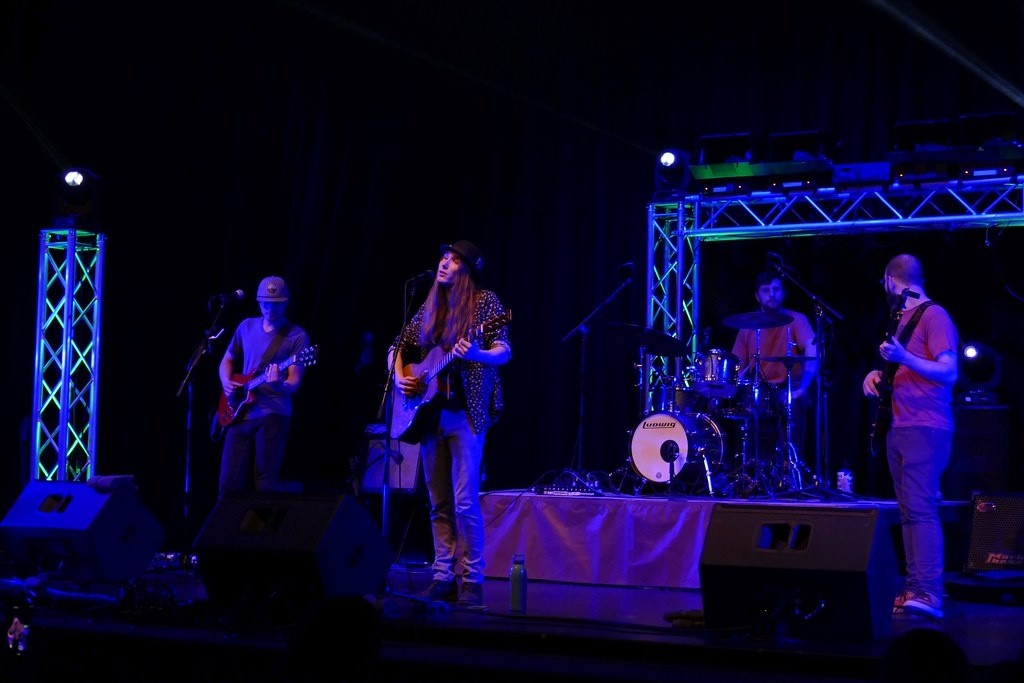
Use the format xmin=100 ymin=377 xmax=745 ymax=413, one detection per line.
xmin=389 ymin=307 xmax=516 ymax=446
xmin=217 ymin=344 xmax=318 ymax=426
xmin=867 ymin=287 xmax=916 ymax=460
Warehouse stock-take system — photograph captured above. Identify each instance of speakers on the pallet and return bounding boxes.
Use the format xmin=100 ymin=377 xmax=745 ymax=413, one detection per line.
xmin=0 ymin=477 xmax=163 ymax=584
xmin=191 ymin=487 xmax=393 ymax=621
xmin=961 ymin=491 xmax=1024 ymax=574
xmin=699 ymin=500 xmax=902 ymax=641
xmin=359 ymin=434 xmax=428 ymax=495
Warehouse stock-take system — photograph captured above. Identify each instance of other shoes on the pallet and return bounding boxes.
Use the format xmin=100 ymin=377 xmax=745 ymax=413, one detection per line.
xmin=893 ymin=589 xmax=955 ymax=621
xmin=419 ymin=577 xmax=455 ymax=598
xmin=456 ymin=581 xmax=484 ymax=604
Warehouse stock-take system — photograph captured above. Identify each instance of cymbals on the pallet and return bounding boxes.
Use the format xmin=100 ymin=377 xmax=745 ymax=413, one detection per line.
xmin=724 ymin=309 xmax=794 ymax=330
xmin=608 ymin=320 xmax=690 ymax=357
xmin=757 ymin=354 xmax=818 ymax=363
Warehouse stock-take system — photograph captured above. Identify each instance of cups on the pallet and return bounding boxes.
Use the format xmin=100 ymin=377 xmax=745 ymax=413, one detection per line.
xmin=836 ymin=468 xmax=854 ymax=494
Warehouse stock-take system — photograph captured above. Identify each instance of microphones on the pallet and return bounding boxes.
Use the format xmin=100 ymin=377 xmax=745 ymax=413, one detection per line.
xmin=667 ymin=444 xmax=676 ymax=460
xmin=406 ymin=270 xmax=435 ymax=283
xmin=381 ymin=443 xmax=404 ymax=465
xmin=212 ymin=290 xmax=244 ymax=300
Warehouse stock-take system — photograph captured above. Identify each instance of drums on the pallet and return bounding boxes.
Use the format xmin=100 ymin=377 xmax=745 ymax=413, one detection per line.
xmin=694 ymin=350 xmax=743 ymax=401
xmin=628 ymin=410 xmax=723 ymax=488
xmin=661 ymin=386 xmax=703 ymax=414
xmin=719 ymin=378 xmax=760 ymax=419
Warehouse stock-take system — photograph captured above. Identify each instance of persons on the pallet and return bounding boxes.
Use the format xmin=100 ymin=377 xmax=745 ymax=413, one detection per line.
xmin=730 ymin=270 xmax=826 ymax=485
xmin=862 ymin=253 xmax=959 ymax=619
xmin=218 ymin=276 xmax=311 ymax=500
xmin=879 ymin=629 xmax=972 ymax=683
xmin=386 ymin=239 xmax=513 ymax=612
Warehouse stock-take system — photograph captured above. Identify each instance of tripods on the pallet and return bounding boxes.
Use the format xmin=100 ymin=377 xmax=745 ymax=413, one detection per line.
xmin=695 ymin=318 xmax=859 ymax=503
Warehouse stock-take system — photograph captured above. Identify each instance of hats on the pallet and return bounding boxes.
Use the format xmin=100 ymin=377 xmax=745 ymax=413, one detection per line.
xmin=439 ymin=240 xmax=484 ymax=274
xmin=257 ymin=275 xmax=289 ymax=302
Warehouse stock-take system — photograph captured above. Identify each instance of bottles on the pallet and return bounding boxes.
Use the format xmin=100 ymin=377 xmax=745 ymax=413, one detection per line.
xmin=508 ymin=555 xmax=527 ymax=611
xmin=5 ymin=604 xmax=33 ymax=657
